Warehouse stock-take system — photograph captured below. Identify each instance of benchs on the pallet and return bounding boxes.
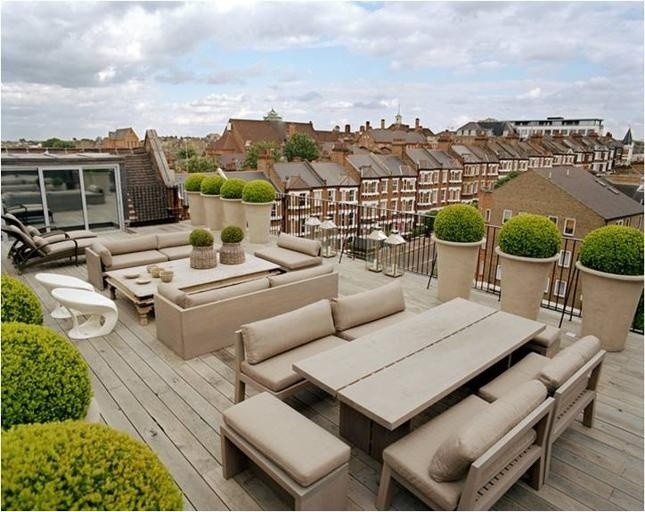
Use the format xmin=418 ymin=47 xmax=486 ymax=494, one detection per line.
xmin=216 ymin=390 xmax=353 ymax=511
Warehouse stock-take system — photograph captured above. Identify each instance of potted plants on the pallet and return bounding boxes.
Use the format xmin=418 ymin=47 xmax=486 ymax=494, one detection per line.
xmin=199 ymin=175 xmax=228 ymax=232
xmin=428 ymin=202 xmax=487 ymax=305
xmin=240 ymin=179 xmax=278 ymax=243
xmin=189 ymin=228 xmax=218 ymax=269
xmin=219 ymin=178 xmax=247 ymax=235
xmin=573 ymin=224 xmax=643 ymax=353
xmin=183 ymin=171 xmax=209 ymax=229
xmin=492 ymin=213 xmax=562 ymax=323
xmin=219 ymin=225 xmax=246 ymax=266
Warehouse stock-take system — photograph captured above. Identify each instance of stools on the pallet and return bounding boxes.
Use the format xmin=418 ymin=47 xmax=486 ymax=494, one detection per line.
xmin=50 ymin=287 xmax=120 ymax=341
xmin=34 ymin=272 xmax=95 ymax=319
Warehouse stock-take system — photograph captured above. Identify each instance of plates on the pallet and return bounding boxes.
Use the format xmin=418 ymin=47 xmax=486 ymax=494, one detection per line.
xmin=124 ymin=270 xmax=141 ymax=279
xmin=135 ymin=277 xmax=152 ymax=285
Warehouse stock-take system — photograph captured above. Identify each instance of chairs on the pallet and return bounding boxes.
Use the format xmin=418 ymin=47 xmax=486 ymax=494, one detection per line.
xmin=1 ymin=212 xmax=65 ymax=265
xmin=1 ymin=224 xmax=97 ymax=276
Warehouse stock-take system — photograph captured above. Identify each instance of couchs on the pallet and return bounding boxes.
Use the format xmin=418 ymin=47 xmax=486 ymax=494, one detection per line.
xmin=85 ymin=227 xmax=212 ymax=291
xmin=477 ymin=334 xmax=607 ymax=486
xmin=232 ymin=279 xmax=419 ymax=406
xmin=376 ymin=395 xmax=556 ymax=511
xmin=253 ymin=232 xmax=325 ymax=273
xmin=153 ymin=262 xmax=340 ymax=362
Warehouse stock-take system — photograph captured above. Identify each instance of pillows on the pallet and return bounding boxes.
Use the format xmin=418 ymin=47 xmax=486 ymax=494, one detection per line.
xmin=157 ymin=280 xmax=193 ymax=309
xmin=427 ymin=380 xmax=546 ymax=485
xmin=276 ymin=232 xmax=323 ymax=258
xmin=185 ymin=274 xmax=270 ymax=309
xmin=239 ymin=298 xmax=338 ymax=366
xmin=99 ymin=233 xmax=157 ymax=255
xmin=329 ymin=278 xmax=407 ymax=332
xmin=91 ymin=238 xmax=113 ymax=268
xmin=265 ymin=261 xmax=334 ymax=288
xmin=157 ymin=227 xmax=211 ymax=250
xmin=531 ymin=334 xmax=602 ymax=398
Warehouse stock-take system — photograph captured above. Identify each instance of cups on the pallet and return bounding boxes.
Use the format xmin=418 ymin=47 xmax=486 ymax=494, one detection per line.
xmin=146 ymin=264 xmax=157 ymax=272
xmin=159 ymin=270 xmax=174 ymax=282
xmin=151 ymin=267 xmax=165 ymax=278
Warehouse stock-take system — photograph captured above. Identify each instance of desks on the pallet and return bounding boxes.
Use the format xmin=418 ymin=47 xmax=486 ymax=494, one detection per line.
xmin=291 ymin=296 xmax=549 ymax=465
xmin=101 ymin=247 xmax=282 ymax=327
